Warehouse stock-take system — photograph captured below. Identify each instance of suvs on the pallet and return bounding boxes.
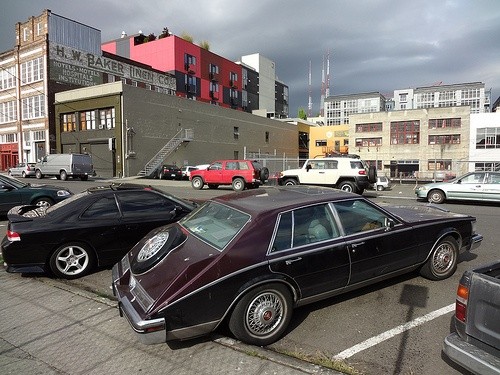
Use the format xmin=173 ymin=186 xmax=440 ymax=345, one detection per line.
xmin=154 ymin=164 xmax=183 ymax=181
xmin=276 ymin=151 xmax=378 ymax=195
xmin=189 ymin=159 xmax=270 ymax=192
xmin=180 ymin=166 xmax=197 ymax=181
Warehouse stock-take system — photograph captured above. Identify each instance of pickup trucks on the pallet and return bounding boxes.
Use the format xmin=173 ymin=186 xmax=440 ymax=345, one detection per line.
xmin=442 ymin=262 xmax=500 ymax=375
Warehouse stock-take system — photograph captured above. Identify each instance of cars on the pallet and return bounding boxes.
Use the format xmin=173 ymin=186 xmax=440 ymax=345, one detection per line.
xmin=196 ymin=164 xmax=211 ymax=171
xmin=369 ymin=176 xmax=392 ymax=192
xmin=413 ymin=170 xmax=500 ymax=205
xmin=0 ymin=173 xmax=75 ymax=221
xmin=7 ymin=162 xmax=45 ymax=178
xmin=110 ymin=184 xmax=483 ymax=347
xmin=0 ymin=182 xmax=230 ymax=280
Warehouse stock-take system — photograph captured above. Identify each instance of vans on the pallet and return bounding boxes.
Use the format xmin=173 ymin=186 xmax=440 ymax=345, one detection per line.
xmin=35 ymin=153 xmax=95 ymax=182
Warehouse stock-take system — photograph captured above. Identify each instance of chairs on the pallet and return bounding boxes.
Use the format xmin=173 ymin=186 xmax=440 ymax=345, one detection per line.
xmin=307 ymin=218 xmax=333 ymax=243
